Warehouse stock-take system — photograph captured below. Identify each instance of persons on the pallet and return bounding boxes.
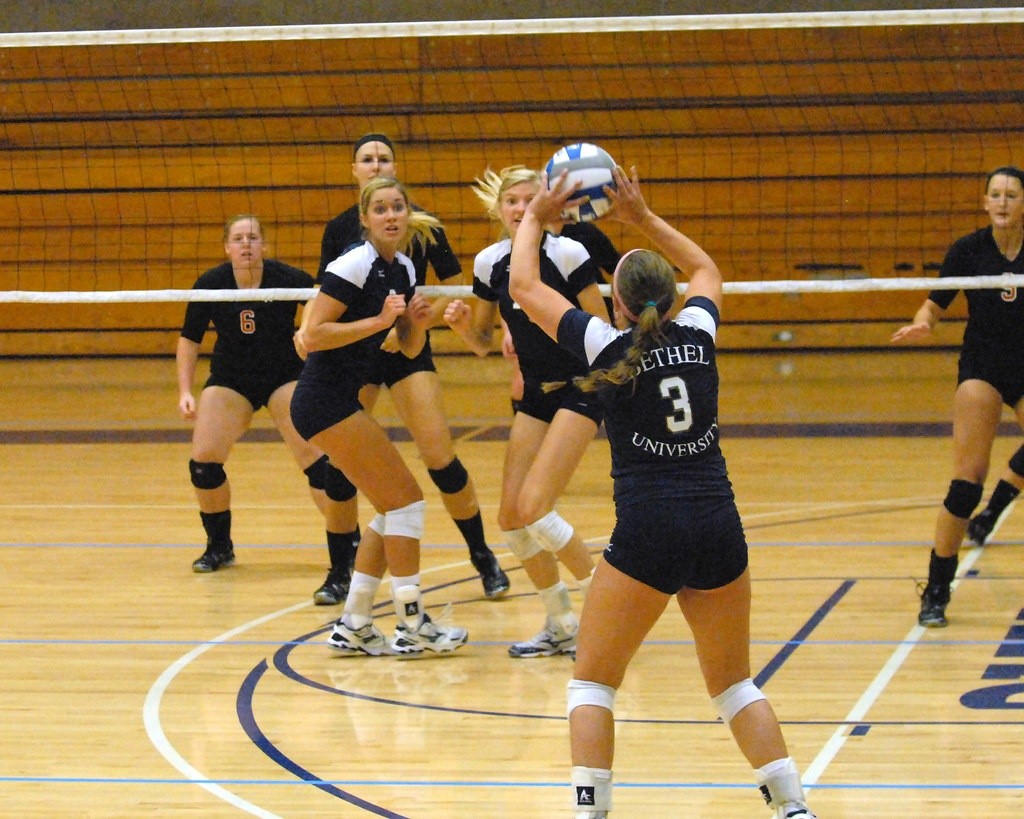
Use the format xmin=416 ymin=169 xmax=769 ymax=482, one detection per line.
xmin=891 ymin=164 xmax=1024 ymax=627
xmin=174 ymin=213 xmax=362 ymax=574
xmin=508 ymin=165 xmax=821 ymax=819
xmin=287 ymin=131 xmax=623 ymax=660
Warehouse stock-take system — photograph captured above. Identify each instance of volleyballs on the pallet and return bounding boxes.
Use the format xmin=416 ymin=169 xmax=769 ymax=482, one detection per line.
xmin=545 ymin=142 xmax=618 ymax=222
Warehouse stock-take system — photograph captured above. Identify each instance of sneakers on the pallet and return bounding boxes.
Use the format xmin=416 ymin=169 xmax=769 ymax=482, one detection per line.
xmin=912 ymin=575 xmax=952 ymax=627
xmin=469 ymin=548 xmax=509 ymax=597
xmin=194 ymin=538 xmax=235 ymax=572
xmin=390 ymin=614 xmax=470 ymax=655
xmin=329 ymin=619 xmax=391 ymax=656
xmin=313 ymin=561 xmax=353 ymax=605
xmin=769 ymin=801 xmax=815 ymax=819
xmin=508 ymin=619 xmax=579 ymax=658
xmin=968 ymin=511 xmax=996 ymax=545
